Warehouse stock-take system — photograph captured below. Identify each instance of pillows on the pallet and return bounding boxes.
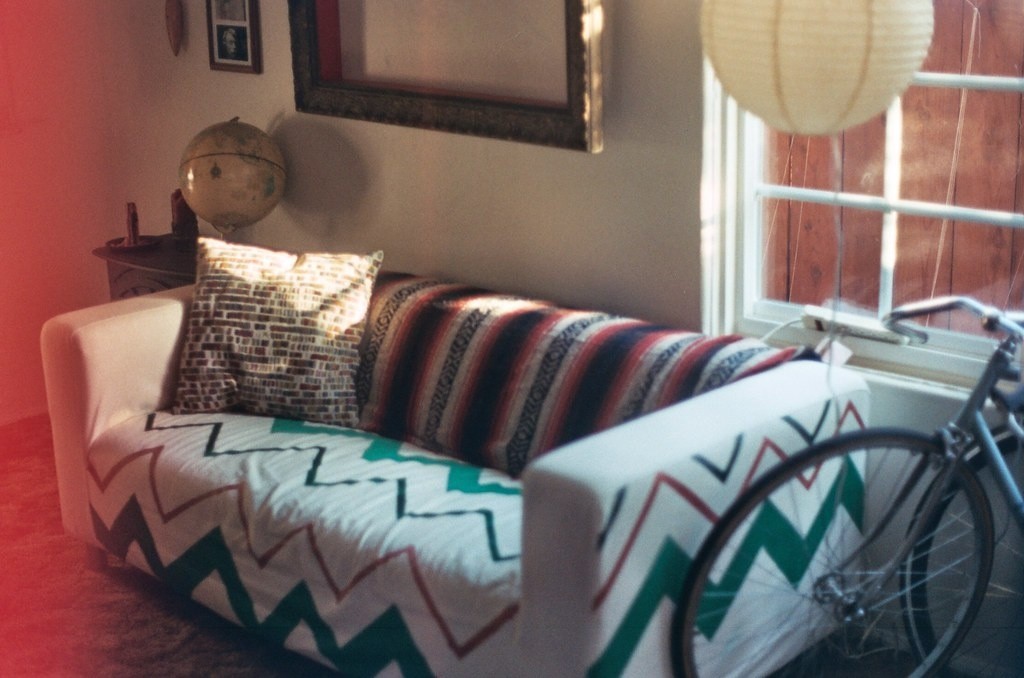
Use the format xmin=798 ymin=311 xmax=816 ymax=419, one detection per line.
xmin=172 ymin=236 xmax=385 ymax=425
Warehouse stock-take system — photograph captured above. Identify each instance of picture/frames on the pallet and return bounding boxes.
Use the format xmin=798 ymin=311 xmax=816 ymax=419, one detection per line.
xmin=206 ymin=0 xmax=260 ymax=74
xmin=288 ymin=1 xmax=602 ymax=155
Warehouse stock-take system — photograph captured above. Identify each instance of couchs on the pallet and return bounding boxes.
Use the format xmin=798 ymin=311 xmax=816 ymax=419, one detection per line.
xmin=40 ymin=272 xmax=867 ymax=678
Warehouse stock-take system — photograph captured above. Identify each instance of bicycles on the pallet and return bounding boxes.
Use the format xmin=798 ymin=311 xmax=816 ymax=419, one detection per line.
xmin=670 ymin=293 xmax=1023 ymax=677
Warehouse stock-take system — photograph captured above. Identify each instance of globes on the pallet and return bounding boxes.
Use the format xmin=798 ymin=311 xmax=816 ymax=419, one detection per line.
xmin=177 ymin=117 xmax=290 ymax=243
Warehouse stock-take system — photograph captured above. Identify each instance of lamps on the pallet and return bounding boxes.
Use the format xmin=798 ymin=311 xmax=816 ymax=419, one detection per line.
xmin=705 ymin=0 xmax=935 ymax=132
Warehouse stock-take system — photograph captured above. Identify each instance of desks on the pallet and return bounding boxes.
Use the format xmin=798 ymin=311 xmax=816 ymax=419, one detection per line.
xmin=94 ymin=233 xmax=197 ymax=301
